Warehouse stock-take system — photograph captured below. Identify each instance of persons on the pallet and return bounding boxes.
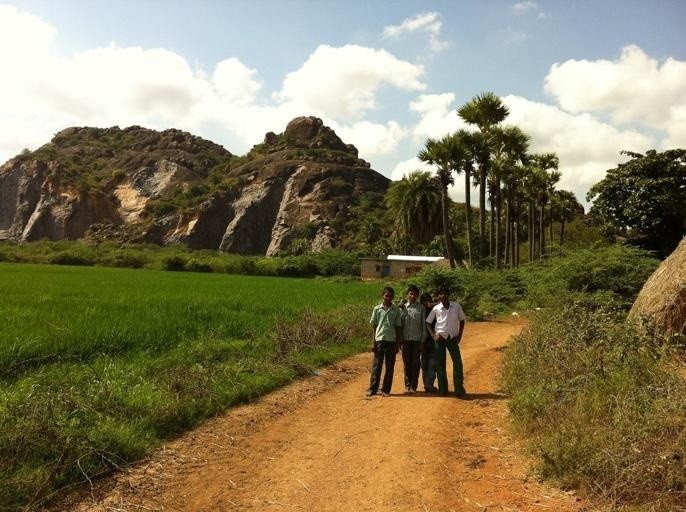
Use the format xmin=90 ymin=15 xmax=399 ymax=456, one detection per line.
xmin=428 ymin=285 xmax=467 ymax=400
xmin=398 ymin=292 xmax=436 ymax=395
xmin=366 ymin=287 xmax=403 ymax=396
xmin=397 ymin=285 xmax=427 ymax=398
